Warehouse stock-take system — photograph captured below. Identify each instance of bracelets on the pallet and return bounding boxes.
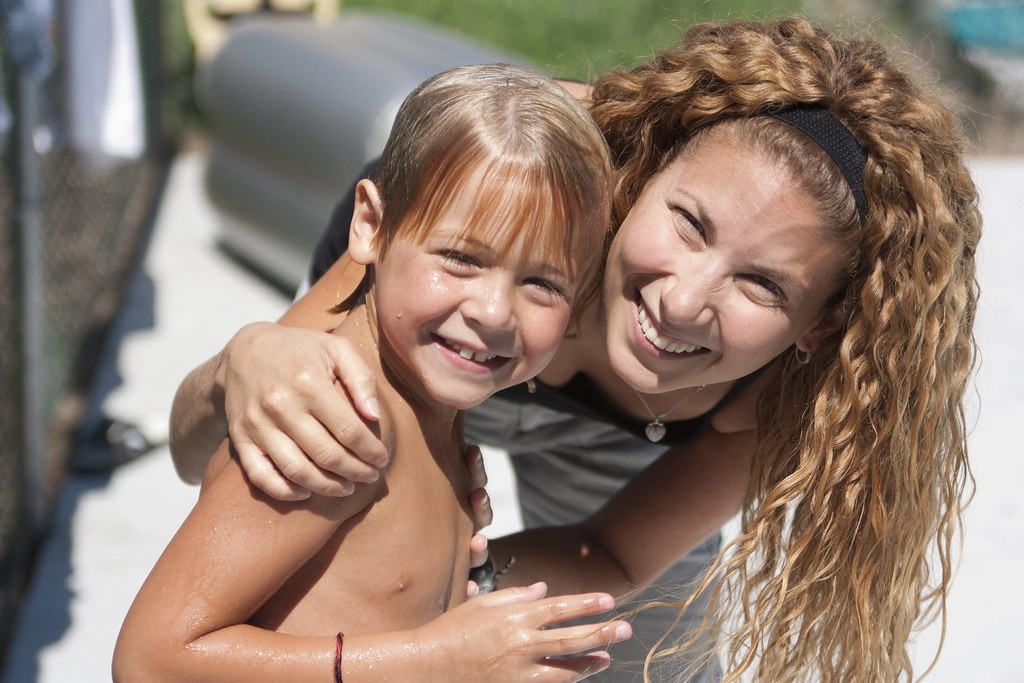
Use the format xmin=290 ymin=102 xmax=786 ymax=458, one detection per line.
xmin=334 ymin=631 xmax=349 ymax=683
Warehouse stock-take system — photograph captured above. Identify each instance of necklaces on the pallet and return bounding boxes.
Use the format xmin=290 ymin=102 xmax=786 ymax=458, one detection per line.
xmin=640 ymin=382 xmax=707 ymax=442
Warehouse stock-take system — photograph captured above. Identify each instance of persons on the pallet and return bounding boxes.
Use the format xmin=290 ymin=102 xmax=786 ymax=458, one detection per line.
xmin=169 ymin=14 xmax=983 ymax=683
xmin=110 ymin=64 xmax=631 ymax=683
